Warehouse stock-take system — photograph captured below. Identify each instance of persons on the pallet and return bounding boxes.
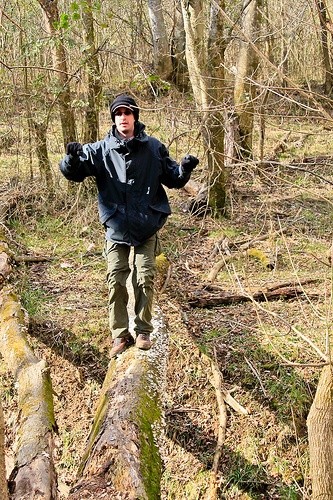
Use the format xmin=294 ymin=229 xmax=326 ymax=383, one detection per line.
xmin=59 ymin=96 xmax=199 ymax=358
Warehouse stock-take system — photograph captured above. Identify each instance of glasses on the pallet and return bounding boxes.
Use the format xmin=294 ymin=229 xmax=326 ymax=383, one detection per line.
xmin=114 ymin=111 xmax=132 ymax=116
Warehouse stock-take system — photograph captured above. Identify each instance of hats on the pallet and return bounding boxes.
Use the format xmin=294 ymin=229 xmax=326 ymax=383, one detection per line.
xmin=110 ymin=95 xmax=140 ymax=123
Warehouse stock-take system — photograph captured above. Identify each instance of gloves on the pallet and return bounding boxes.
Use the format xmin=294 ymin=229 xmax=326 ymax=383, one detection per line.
xmin=180 ymin=155 xmax=200 ymax=171
xmin=66 ymin=142 xmax=84 ymax=157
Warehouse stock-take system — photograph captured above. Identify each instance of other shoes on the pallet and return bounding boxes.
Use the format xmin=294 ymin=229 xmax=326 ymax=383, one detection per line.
xmin=108 ymin=332 xmax=135 ymax=358
xmin=135 ymin=332 xmax=151 ymax=350
